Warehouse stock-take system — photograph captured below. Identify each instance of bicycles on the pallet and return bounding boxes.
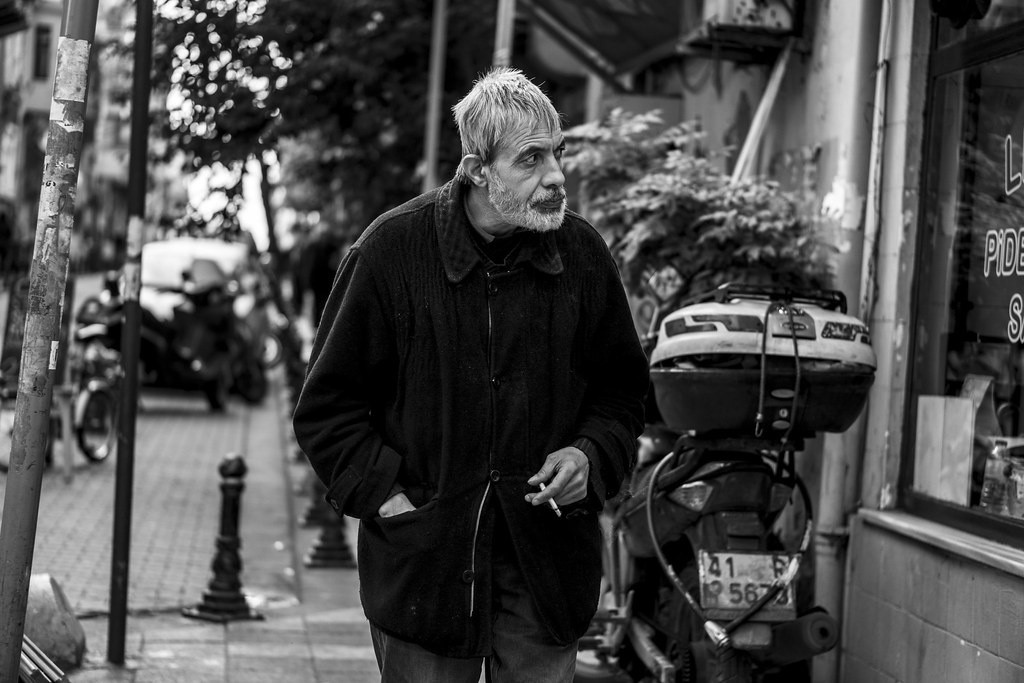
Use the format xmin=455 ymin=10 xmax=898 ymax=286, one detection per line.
xmin=0 ymin=320 xmax=117 ymax=472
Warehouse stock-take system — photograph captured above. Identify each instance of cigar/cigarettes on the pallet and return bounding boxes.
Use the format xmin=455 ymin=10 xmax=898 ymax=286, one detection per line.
xmin=539 ymin=483 xmax=561 ymax=518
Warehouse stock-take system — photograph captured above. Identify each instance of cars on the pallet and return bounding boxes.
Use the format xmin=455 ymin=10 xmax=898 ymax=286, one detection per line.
xmin=111 ymin=236 xmax=283 ymax=374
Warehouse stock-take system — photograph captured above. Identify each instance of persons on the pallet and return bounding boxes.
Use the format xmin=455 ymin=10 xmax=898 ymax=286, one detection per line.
xmin=291 ymin=206 xmax=347 ymax=345
xmin=293 ymin=64 xmax=654 ymax=683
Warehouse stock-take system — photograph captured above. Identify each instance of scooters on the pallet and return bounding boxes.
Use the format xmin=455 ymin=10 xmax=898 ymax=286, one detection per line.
xmin=79 ymin=256 xmax=268 ymax=414
xmin=572 ymin=279 xmax=877 ymax=683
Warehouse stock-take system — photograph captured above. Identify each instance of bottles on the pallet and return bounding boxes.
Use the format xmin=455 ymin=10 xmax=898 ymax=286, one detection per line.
xmin=979 ymin=440 xmax=1011 ymax=516
